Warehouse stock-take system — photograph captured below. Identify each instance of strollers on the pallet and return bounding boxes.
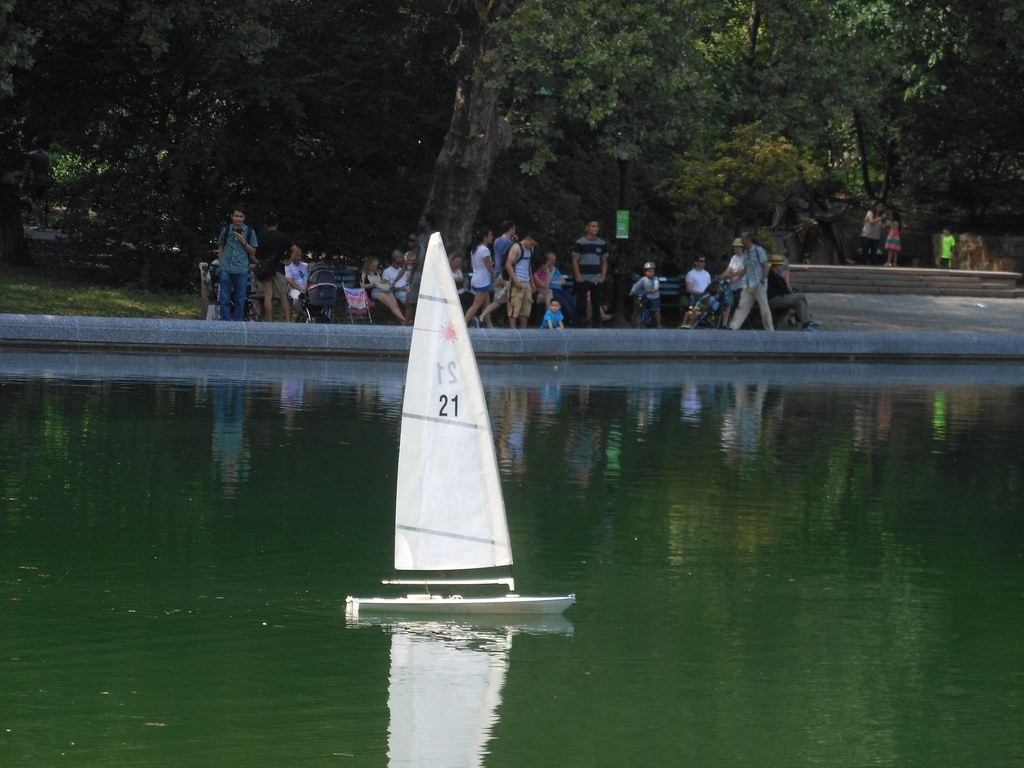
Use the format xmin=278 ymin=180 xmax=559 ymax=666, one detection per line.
xmin=298 ymin=266 xmax=337 ymax=323
xmin=678 ymin=275 xmax=731 ymax=330
xmin=206 ymin=259 xmax=259 ymax=322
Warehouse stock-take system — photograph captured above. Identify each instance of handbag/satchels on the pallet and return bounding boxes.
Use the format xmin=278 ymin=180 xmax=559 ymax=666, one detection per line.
xmin=255 ymin=255 xmax=277 ymax=281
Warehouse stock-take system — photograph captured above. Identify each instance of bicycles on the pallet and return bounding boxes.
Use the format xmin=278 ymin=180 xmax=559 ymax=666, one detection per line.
xmin=629 ymin=291 xmax=652 ymax=328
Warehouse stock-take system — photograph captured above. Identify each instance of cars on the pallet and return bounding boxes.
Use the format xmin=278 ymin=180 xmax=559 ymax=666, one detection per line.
xmin=622 ymin=260 xmax=687 ymax=318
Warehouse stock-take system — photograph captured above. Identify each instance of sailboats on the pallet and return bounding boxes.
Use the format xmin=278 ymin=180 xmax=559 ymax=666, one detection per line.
xmin=346 ymin=231 xmax=576 ymax=616
xmin=351 ymin=620 xmax=575 ymax=768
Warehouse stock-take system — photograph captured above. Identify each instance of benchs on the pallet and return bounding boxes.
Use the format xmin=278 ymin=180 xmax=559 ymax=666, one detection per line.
xmin=653 ymin=276 xmax=690 ymax=308
xmin=336 ymin=269 xmax=359 ymax=300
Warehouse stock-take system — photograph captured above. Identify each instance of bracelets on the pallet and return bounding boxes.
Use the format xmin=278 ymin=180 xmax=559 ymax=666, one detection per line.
xmin=242 ymin=242 xmax=247 ymax=245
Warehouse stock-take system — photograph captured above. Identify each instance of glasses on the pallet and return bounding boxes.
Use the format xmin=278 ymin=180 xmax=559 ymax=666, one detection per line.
xmin=696 ymin=259 xmax=707 ymax=263
xmin=407 ymin=237 xmax=418 ymax=242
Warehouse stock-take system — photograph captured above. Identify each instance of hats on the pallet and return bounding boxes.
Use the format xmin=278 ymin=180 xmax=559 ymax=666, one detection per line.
xmin=732 ymin=238 xmax=744 ymax=247
xmin=769 ymin=253 xmax=785 ymax=266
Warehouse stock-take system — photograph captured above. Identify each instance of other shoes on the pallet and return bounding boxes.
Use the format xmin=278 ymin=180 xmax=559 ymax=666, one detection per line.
xmin=802 ymin=321 xmax=821 ymax=330
xmin=602 ymin=313 xmax=613 ymax=321
xmin=682 ymin=323 xmax=693 ymax=329
xmin=474 ymin=315 xmax=483 ymax=329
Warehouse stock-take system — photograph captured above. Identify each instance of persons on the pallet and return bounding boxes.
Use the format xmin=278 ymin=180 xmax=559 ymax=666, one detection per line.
xmin=463 ymin=227 xmax=494 ymax=328
xmin=939 ymin=227 xmax=956 ymax=268
xmin=767 ymin=254 xmax=823 ymax=332
xmin=628 ymin=262 xmax=664 ymax=327
xmin=506 ymin=232 xmax=538 ymax=328
xmin=250 ymin=215 xmax=298 ymax=322
xmin=883 ymin=209 xmax=902 ymax=267
xmin=218 ymin=205 xmax=258 ymax=321
xmin=686 ymin=253 xmax=731 ymax=330
xmin=534 ymin=250 xmax=577 ymax=328
xmin=571 ymin=220 xmax=611 ymax=328
xmin=719 ymin=237 xmax=747 ymax=316
xmin=449 ymin=251 xmax=475 ymax=315
xmin=858 ymin=201 xmax=885 ymax=266
xmin=541 ymin=298 xmax=564 ymax=329
xmin=727 ymin=230 xmax=774 ymax=331
xmin=284 ymin=247 xmax=309 ymax=303
xmin=476 ymin=221 xmax=518 ymax=327
xmin=357 ymin=233 xmax=420 ymax=326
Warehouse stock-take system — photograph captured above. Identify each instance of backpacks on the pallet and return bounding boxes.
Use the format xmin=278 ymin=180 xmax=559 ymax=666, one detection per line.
xmin=500 ymin=239 xmax=524 ymax=281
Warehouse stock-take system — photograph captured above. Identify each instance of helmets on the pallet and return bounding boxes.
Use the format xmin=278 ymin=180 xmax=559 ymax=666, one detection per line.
xmin=644 ymin=262 xmax=657 ymax=273
